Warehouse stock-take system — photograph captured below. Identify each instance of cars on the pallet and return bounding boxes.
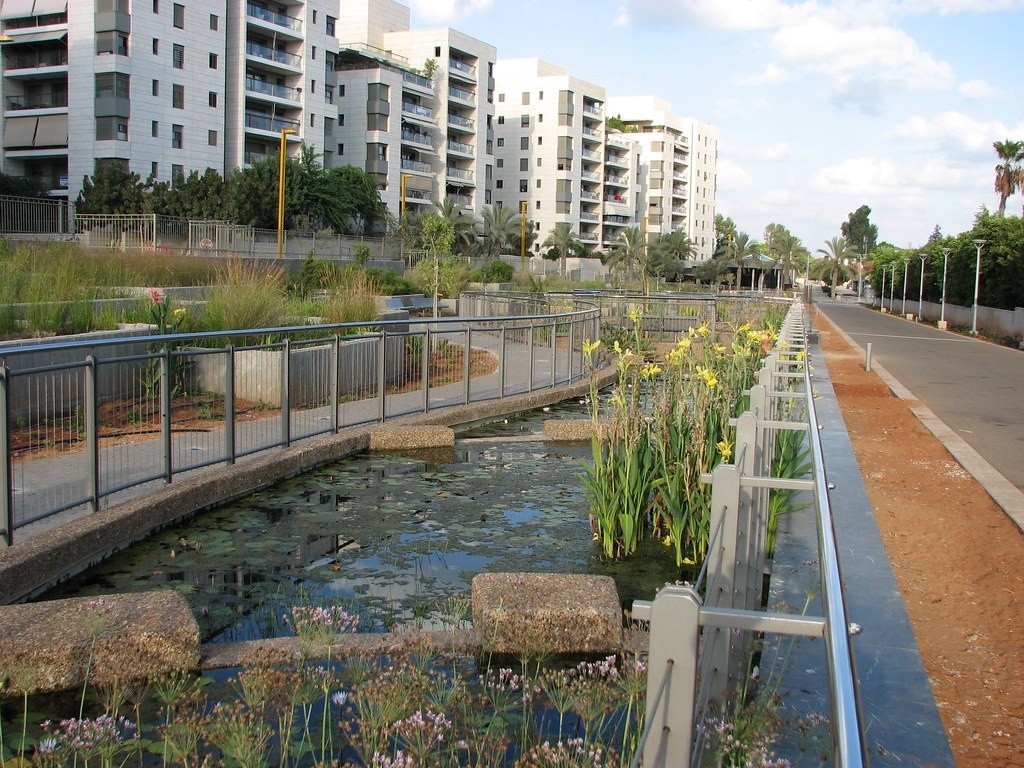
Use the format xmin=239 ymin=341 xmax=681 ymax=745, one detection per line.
xmin=821 ymin=283 xmax=831 ymax=289
xmin=797 ymin=278 xmax=806 ymax=284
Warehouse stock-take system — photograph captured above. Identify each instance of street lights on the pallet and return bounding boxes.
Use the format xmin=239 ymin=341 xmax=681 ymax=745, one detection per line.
xmin=522 ymin=202 xmax=531 ymax=259
xmin=881 ymin=265 xmax=888 ymax=312
xmin=278 ymin=129 xmax=297 ymax=259
xmin=970 ymin=239 xmax=987 ymax=335
xmin=918 ymin=254 xmax=928 ymax=321
xmin=642 ymin=217 xmax=649 ymax=254
xmin=890 ymin=261 xmax=898 ymax=314
xmin=402 ymin=174 xmax=414 ymax=233
xmin=902 ymin=258 xmax=911 ymax=315
xmin=937 ymin=248 xmax=953 ymax=329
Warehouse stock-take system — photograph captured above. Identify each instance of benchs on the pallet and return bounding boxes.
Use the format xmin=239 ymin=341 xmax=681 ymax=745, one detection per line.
xmin=411 ymin=298 xmax=450 ymax=317
xmin=384 ymin=298 xmax=414 ymax=310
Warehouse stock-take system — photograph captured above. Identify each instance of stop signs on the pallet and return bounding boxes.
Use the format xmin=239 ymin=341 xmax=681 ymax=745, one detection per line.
xmin=199 ymin=239 xmax=213 ymax=249
